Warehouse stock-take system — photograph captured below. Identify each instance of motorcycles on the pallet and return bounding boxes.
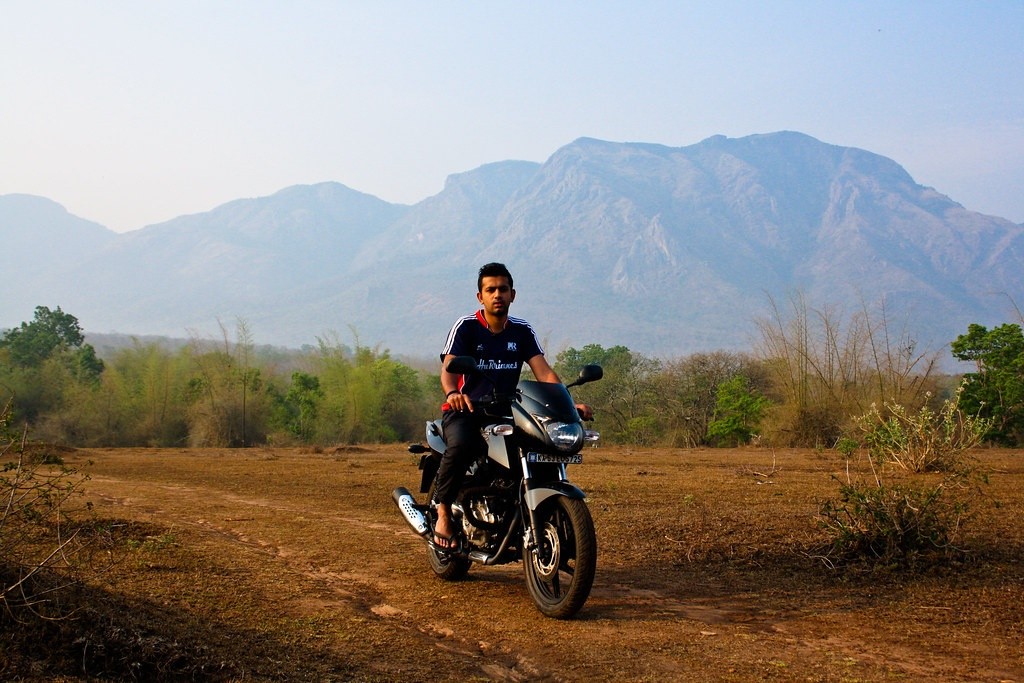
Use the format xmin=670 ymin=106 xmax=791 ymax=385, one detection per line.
xmin=391 ymin=364 xmax=603 ymax=618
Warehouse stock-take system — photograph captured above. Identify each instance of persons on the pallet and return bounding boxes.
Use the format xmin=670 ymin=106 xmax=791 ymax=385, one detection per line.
xmin=432 ymin=261 xmax=594 ymax=554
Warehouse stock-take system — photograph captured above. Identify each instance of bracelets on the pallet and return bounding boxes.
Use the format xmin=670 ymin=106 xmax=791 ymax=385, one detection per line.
xmin=446 ymin=390 xmax=461 ymax=399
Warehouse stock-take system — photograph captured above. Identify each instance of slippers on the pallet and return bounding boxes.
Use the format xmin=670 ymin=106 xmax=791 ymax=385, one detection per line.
xmin=430 ymin=521 xmax=460 ymax=552
